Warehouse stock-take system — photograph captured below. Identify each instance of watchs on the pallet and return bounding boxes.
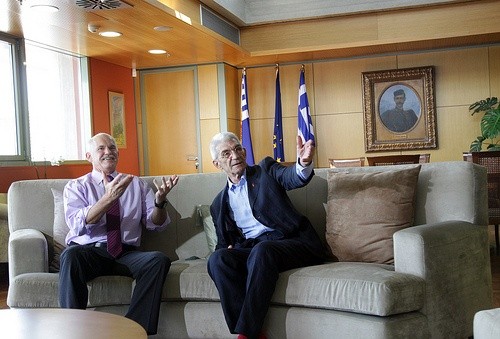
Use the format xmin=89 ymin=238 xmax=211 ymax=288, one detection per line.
xmin=154 ymin=199 xmax=168 ymax=208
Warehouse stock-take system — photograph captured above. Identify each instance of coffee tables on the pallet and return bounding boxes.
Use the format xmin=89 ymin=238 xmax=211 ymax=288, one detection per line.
xmin=0 ymin=306 xmax=148 ymax=339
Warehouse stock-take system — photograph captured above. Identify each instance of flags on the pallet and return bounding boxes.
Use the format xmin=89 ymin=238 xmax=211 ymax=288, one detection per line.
xmin=273 ymin=71 xmax=284 ymax=163
xmin=241 ymin=77 xmax=255 ymax=165
xmin=297 ymin=72 xmax=315 ymax=146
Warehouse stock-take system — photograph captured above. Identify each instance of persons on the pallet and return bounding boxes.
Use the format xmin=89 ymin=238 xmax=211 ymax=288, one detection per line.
xmin=57 ymin=134 xmax=179 ymax=335
xmin=207 ymin=132 xmax=317 ymax=339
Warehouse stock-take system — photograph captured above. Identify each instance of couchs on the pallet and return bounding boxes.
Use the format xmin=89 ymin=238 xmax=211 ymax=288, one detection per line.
xmin=7 ymin=160 xmax=495 ymax=339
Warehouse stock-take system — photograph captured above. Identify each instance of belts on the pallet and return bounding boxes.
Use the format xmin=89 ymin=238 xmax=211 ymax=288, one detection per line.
xmin=90 ymin=242 xmax=127 ymax=247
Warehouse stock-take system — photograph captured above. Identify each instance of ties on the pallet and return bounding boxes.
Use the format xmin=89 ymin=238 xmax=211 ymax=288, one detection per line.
xmin=105 ymin=177 xmax=123 ymax=258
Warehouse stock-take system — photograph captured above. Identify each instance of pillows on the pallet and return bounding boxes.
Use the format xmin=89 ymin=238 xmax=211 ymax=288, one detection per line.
xmin=50 ymin=187 xmax=69 ymax=272
xmin=326 ymin=164 xmax=422 ymax=266
xmin=196 ymin=204 xmax=234 ymax=259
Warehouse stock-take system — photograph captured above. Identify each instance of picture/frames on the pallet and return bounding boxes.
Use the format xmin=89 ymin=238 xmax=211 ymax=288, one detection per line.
xmin=109 ymin=91 xmax=127 ymax=148
xmin=361 ymin=65 xmax=438 ymax=153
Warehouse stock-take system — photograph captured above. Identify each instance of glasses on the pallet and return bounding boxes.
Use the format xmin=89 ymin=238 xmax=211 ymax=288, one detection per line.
xmin=219 ymin=147 xmax=246 ymax=158
